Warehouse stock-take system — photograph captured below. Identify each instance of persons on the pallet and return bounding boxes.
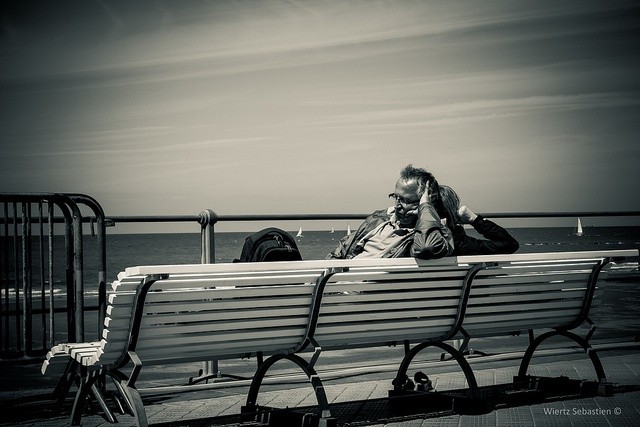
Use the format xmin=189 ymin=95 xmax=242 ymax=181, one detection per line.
xmin=434 ymin=185 xmax=520 ymax=255
xmin=304 ymin=163 xmax=454 ymax=307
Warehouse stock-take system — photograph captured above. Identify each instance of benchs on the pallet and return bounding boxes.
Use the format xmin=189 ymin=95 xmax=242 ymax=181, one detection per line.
xmin=40 ymin=250 xmax=639 ymax=427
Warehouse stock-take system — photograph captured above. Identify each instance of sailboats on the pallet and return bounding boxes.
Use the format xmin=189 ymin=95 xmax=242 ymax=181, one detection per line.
xmin=347 ymin=225 xmax=352 ymax=236
xmin=329 ymin=226 xmax=335 ymax=233
xmin=575 ymin=218 xmax=583 ymax=237
xmin=296 ymin=227 xmax=303 ymax=237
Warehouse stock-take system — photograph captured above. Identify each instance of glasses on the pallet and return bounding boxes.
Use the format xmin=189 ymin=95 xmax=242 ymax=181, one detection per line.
xmin=389 ymin=192 xmax=420 ymax=204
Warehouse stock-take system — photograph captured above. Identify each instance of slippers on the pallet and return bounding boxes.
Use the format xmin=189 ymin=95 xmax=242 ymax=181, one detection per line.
xmin=413 ymin=370 xmax=432 ymax=391
xmin=392 ymin=374 xmax=414 ymax=390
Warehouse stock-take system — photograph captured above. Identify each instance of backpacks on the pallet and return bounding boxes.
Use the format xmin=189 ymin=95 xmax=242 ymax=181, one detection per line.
xmin=233 ymin=227 xmax=305 ymax=300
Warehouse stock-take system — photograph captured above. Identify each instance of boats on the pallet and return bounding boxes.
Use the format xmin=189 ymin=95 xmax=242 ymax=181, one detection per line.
xmin=1 ymin=288 xmax=61 ymax=295
